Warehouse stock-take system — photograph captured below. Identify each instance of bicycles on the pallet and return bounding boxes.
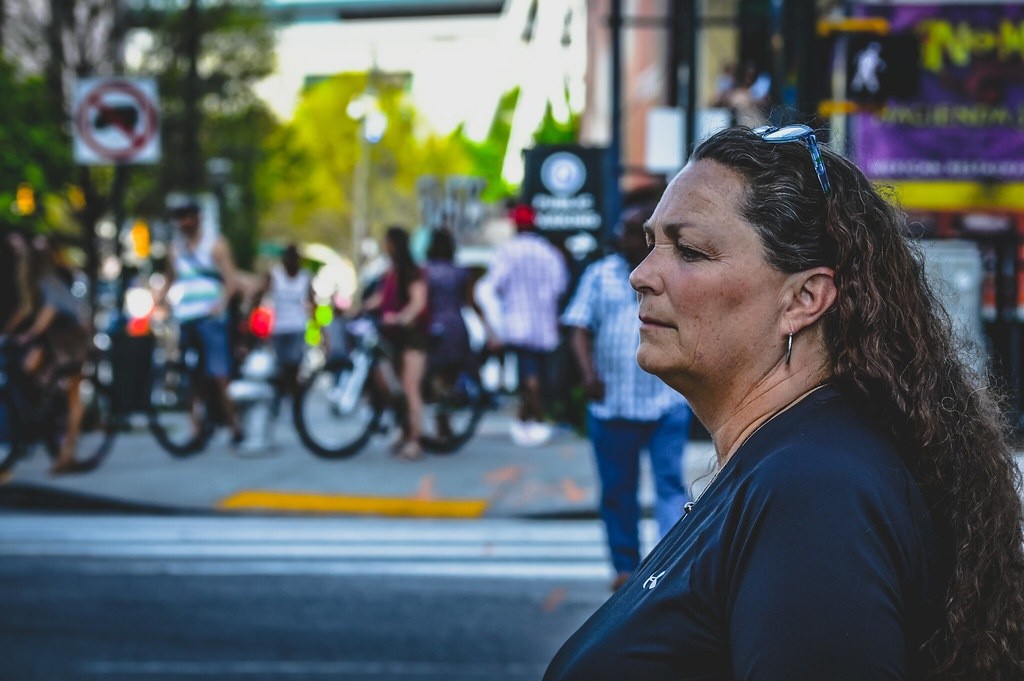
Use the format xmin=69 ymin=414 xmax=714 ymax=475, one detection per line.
xmin=0 ymin=302 xmax=220 ymax=478
xmin=293 ymin=304 xmax=484 ymax=452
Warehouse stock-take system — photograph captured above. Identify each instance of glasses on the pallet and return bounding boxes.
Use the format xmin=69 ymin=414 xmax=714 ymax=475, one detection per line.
xmin=752 ymin=124 xmax=830 ymax=198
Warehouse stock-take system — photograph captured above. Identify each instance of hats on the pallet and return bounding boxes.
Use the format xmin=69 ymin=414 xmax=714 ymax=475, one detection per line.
xmin=164 ymin=192 xmax=201 ymax=218
xmin=508 ymin=205 xmax=534 ymax=224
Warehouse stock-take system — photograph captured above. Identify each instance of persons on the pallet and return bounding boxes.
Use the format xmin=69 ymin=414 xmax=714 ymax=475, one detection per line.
xmin=422 ymin=227 xmax=505 ymax=443
xmin=0 ymin=224 xmax=92 ymax=476
xmin=345 ymin=227 xmax=431 ymax=461
xmin=226 ymin=289 xmax=251 ymax=364
xmin=559 ymin=189 xmax=692 ymax=591
xmin=154 ymin=204 xmax=246 ymax=447
xmin=543 ymin=118 xmax=1024 ymax=680
xmin=473 ymin=230 xmax=570 ymax=447
xmin=248 ymin=245 xmax=314 ymax=452
xmin=325 ymin=308 xmax=348 ymax=367
